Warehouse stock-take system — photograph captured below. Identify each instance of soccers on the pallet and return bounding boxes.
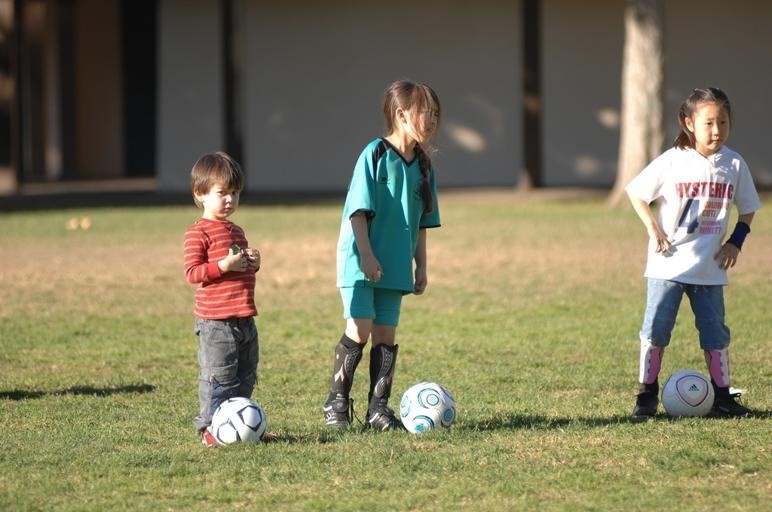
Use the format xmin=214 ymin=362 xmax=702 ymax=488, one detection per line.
xmin=662 ymin=368 xmax=715 ymax=416
xmin=400 ymin=381 xmax=455 ymax=433
xmin=211 ymin=395 xmax=266 ymax=446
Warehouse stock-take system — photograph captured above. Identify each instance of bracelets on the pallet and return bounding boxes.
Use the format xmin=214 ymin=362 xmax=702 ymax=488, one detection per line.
xmin=727 ymin=221 xmax=751 ymax=250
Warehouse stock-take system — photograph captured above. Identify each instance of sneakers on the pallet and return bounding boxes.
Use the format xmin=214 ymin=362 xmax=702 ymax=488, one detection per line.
xmin=365 ymin=407 xmax=406 ymax=432
xmin=711 ymin=393 xmax=752 ymax=417
xmin=323 ymin=399 xmax=362 ymax=426
xmin=633 ymin=391 xmax=658 ymax=415
xmin=202 ymin=426 xmax=218 ymax=448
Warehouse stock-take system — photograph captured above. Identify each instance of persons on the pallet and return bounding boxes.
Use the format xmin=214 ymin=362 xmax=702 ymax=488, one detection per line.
xmin=184 ymin=152 xmax=274 ymax=445
xmin=323 ymin=81 xmax=441 ymax=431
xmin=623 ymin=87 xmax=761 ymax=417
